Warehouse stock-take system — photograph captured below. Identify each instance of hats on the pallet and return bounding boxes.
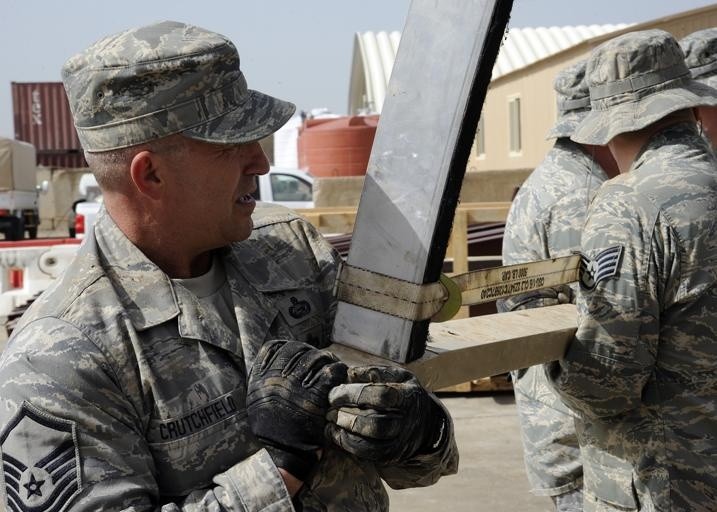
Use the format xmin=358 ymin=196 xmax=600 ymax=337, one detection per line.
xmin=61 ymin=21 xmax=296 ymax=154
xmin=546 ymin=28 xmax=716 ymax=147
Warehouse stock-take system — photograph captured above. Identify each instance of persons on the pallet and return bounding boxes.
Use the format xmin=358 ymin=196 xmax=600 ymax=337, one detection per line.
xmin=495 ymin=27 xmax=717 ymax=512
xmin=1 ymin=19 xmax=459 ymax=512
xmin=676 ymin=26 xmax=717 ymax=162
xmin=500 ymin=51 xmax=620 ymax=511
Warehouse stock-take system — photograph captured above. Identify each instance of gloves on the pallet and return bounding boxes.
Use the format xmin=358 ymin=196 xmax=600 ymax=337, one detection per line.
xmin=503 ymin=283 xmax=572 ymax=312
xmin=246 ymin=339 xmax=426 ymax=468
